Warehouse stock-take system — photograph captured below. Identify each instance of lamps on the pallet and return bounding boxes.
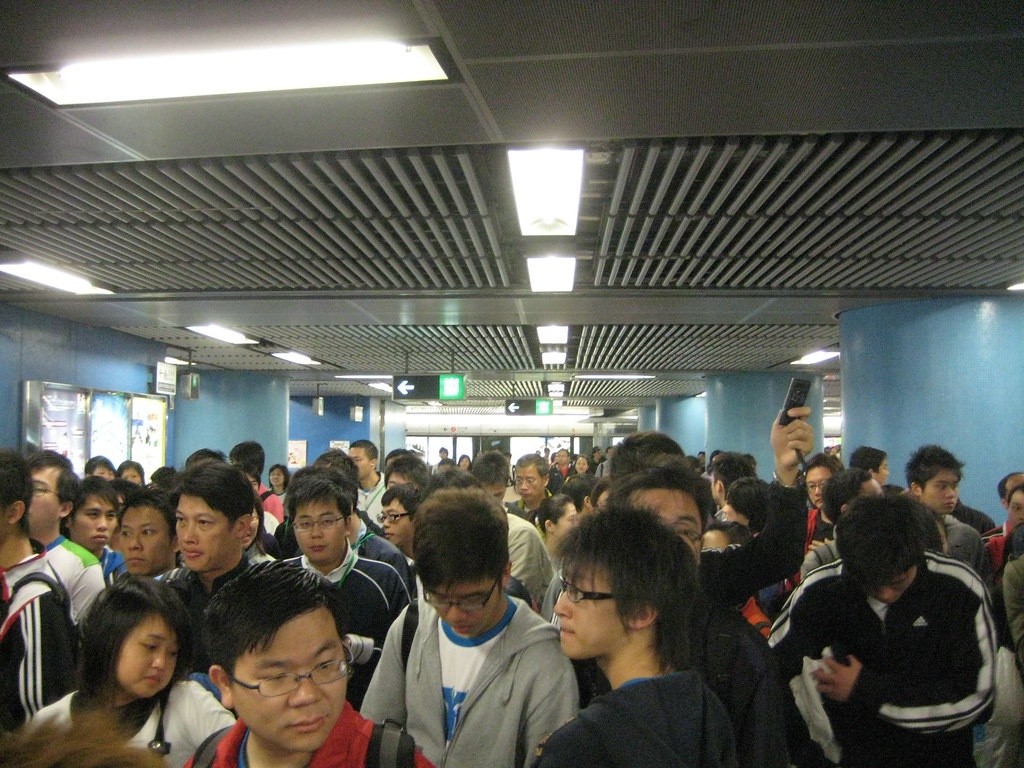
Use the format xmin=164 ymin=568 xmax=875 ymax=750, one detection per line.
xmin=789 ymin=345 xmax=840 ymax=365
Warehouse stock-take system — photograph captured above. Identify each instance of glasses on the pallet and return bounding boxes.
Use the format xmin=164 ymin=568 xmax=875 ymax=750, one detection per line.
xmin=32 ymin=480 xmax=60 ymax=498
xmin=422 ymin=573 xmax=499 ymax=615
xmin=558 ymin=575 xmax=618 ymax=603
xmin=802 ymin=478 xmax=828 ymax=494
xmin=290 ymin=516 xmax=347 ymax=532
xmin=232 ymin=638 xmax=355 ymax=698
xmin=513 ymin=475 xmax=544 ymax=486
xmin=377 ymin=512 xmax=415 ymax=524
xmin=669 ymin=520 xmax=703 ymax=544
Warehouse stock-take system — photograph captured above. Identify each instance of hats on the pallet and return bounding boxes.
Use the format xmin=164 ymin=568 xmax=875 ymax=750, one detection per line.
xmin=591 ymin=446 xmax=600 ymax=454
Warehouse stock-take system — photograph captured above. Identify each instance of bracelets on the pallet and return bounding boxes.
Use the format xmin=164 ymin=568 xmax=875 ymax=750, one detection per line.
xmin=771 ymin=468 xmax=801 ymax=492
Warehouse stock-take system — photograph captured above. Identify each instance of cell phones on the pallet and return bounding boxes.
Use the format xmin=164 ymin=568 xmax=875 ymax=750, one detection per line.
xmin=779 ymin=377 xmax=811 ymax=426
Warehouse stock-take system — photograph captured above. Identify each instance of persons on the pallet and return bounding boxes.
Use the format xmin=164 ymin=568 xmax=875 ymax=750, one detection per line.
xmin=183 ymin=557 xmax=432 ymax=768
xmin=360 ymin=486 xmax=577 ymax=768
xmin=24 ymin=574 xmax=236 ymax=767
xmin=535 ymin=505 xmax=736 ymax=767
xmin=768 ymin=494 xmax=995 ymax=767
xmin=0 ymin=408 xmax=1024 ymax=768
